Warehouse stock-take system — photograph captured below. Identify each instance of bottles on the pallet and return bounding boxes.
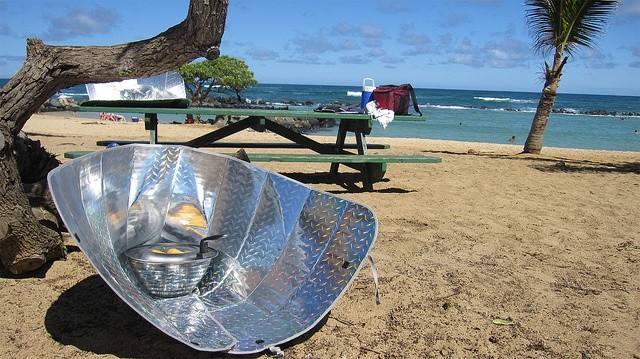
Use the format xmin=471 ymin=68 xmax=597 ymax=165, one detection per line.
xmin=360 ymin=85 xmax=376 ymax=112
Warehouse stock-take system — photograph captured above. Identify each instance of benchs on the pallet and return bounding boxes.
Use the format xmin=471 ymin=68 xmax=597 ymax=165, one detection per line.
xmin=64 ymin=149 xmax=442 ymax=163
xmin=97 ymin=140 xmax=391 ymax=150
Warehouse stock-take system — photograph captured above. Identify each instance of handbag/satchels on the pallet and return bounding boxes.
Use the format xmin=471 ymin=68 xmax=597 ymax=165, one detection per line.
xmin=370 ymin=83 xmax=423 ymax=117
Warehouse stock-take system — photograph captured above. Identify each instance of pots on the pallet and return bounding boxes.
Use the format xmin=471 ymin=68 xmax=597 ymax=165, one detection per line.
xmin=129 ymin=240 xmax=218 ymax=299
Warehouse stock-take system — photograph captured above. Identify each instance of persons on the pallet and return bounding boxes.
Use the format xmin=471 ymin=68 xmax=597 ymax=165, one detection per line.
xmin=633 ymin=129 xmax=639 ymax=135
xmin=98 ymin=112 xmax=127 ymax=122
xmin=507 ymin=135 xmax=516 ymax=142
xmin=459 ymin=122 xmax=462 ymax=125
